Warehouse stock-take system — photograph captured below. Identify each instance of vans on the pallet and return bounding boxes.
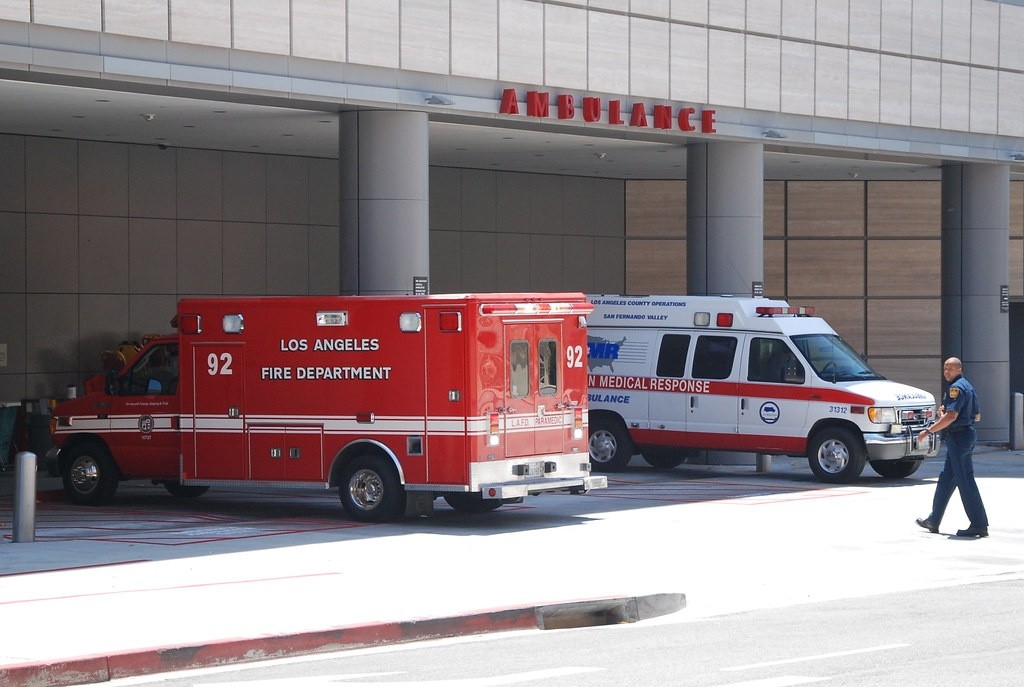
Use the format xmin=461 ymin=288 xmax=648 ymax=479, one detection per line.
xmin=580 ymin=293 xmax=941 ymax=484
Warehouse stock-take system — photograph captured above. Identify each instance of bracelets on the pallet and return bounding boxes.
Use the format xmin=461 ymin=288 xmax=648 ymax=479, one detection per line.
xmin=925 ymin=428 xmax=933 ymax=435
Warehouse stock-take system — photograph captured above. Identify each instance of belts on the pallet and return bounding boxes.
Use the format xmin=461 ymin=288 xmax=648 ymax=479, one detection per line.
xmin=947 ymin=426 xmax=973 ymax=434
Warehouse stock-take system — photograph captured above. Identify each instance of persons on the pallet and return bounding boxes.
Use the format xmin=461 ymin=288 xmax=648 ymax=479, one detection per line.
xmin=915 ymin=357 xmax=989 ymax=539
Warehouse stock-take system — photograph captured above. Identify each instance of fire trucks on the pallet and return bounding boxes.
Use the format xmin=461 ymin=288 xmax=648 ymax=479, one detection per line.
xmin=42 ymin=295 xmax=609 ymax=527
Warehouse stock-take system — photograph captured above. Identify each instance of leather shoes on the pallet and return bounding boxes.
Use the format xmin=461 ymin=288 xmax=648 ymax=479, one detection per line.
xmin=956 ymin=525 xmax=988 ymax=537
xmin=916 ymin=518 xmax=939 ymax=533
